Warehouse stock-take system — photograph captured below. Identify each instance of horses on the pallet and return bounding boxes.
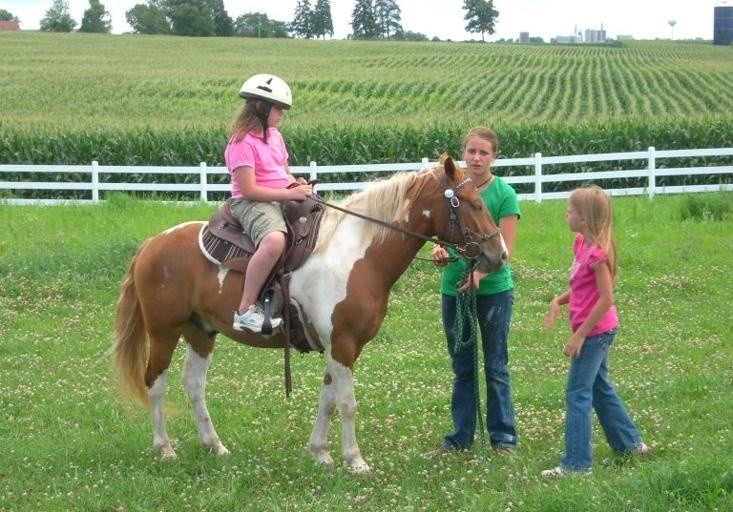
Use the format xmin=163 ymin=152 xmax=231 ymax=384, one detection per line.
xmin=88 ymin=151 xmax=510 ymax=475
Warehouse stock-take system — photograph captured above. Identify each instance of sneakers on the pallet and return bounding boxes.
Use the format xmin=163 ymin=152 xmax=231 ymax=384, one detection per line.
xmin=633 ymin=442 xmax=649 ymax=461
xmin=232 ymin=304 xmax=283 ymax=334
xmin=542 ymin=466 xmax=592 ymax=477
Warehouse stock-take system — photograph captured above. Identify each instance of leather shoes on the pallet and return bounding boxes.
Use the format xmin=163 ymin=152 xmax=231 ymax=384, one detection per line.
xmin=493 ymin=448 xmax=514 ymax=456
xmin=424 ymin=444 xmax=453 ymax=460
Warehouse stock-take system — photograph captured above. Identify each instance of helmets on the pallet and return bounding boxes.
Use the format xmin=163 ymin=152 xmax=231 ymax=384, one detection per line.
xmin=239 ymin=73 xmax=292 ymax=109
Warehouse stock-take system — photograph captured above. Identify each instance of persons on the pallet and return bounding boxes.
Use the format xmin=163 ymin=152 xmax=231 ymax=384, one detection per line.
xmin=431 ymin=124 xmax=523 ymax=458
xmin=540 ymin=183 xmax=648 ymax=481
xmin=222 ymin=69 xmax=315 ymax=336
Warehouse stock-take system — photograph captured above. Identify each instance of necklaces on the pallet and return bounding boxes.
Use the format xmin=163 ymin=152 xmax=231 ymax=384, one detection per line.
xmin=476 ymin=174 xmax=493 ymax=189
